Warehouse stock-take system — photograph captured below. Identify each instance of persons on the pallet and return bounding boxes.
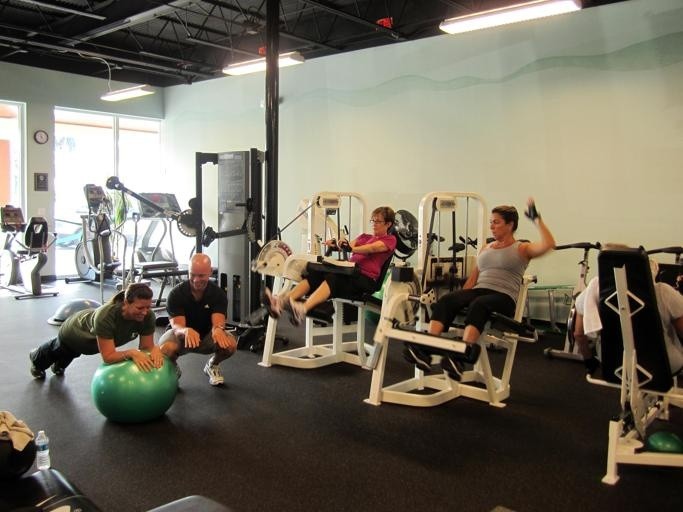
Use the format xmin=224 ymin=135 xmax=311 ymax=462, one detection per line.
xmin=574 ymin=244 xmax=683 ymax=377
xmin=159 ymin=253 xmax=236 ymax=386
xmin=30 ymin=283 xmax=163 ymax=379
xmin=403 ymin=196 xmax=555 ymax=381
xmin=261 ymin=207 xmax=397 ymax=327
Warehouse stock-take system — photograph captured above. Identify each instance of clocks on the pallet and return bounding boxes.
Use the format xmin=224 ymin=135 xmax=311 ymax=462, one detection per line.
xmin=32 ymin=130 xmax=49 ymax=145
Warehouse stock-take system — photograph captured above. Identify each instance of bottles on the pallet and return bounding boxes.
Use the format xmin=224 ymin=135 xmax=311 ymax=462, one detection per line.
xmin=312 ymin=234 xmax=323 ymax=256
xmin=33 ymin=431 xmax=51 ymax=472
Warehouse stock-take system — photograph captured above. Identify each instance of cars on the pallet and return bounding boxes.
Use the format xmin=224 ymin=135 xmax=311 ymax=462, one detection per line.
xmin=55 ymin=209 xmax=89 ymax=249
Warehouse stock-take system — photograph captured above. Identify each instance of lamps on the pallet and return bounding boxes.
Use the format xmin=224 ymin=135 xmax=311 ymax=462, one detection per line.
xmin=99 ymin=82 xmax=156 ymax=102
xmin=438 ymin=0 xmax=583 ymax=36
xmin=220 ymin=49 xmax=305 ymax=77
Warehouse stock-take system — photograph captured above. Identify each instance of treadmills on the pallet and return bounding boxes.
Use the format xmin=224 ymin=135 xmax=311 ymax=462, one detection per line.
xmin=137 ymin=193 xmax=216 ymax=288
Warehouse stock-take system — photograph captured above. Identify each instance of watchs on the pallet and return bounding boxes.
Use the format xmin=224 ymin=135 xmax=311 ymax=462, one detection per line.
xmin=212 ymin=325 xmax=226 ymax=332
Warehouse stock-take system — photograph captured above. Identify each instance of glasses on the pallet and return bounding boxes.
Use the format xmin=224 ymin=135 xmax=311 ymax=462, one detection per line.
xmin=370 ymin=220 xmax=385 ymax=224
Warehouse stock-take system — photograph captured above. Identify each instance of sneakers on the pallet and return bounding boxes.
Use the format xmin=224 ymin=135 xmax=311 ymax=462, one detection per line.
xmin=203 ymin=357 xmax=224 ymax=385
xmin=442 ymin=351 xmax=463 ymax=381
xmin=405 ymin=342 xmax=432 ymax=370
xmin=289 ymin=296 xmax=304 ymax=326
xmin=29 ymin=349 xmax=45 ymax=380
xmin=262 ymin=287 xmax=283 ymax=318
xmin=51 ymin=363 xmax=64 ymax=376
